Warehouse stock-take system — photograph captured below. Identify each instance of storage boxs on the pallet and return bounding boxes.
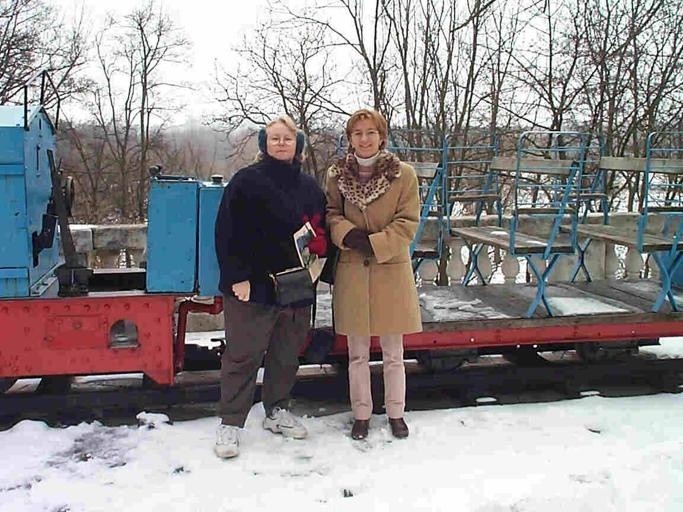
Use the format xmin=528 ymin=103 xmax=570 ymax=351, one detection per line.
xmin=555 ymin=131 xmax=683 ymax=313
xmin=442 ymin=131 xmax=584 ymax=318
xmin=338 ymin=132 xmax=447 ymax=274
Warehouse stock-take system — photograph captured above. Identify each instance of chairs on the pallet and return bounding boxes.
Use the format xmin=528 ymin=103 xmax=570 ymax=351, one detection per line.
xmin=351 ymin=416 xmax=370 ymax=440
xmin=213 ymin=423 xmax=239 ymax=459
xmin=388 ymin=414 xmax=409 ymax=439
xmin=262 ymin=405 xmax=307 ymax=439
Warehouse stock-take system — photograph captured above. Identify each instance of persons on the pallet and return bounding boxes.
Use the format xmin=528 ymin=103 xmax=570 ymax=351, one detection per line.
xmin=214 ymin=117 xmax=339 ymax=457
xmin=321 ymin=108 xmax=423 ymax=440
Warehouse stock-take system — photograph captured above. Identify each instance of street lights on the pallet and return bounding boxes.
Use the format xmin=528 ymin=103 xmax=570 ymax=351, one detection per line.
xmin=272 ymin=265 xmax=315 ymax=308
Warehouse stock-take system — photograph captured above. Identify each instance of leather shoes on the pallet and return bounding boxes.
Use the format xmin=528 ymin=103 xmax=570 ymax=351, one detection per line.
xmin=301 ymin=212 xmax=328 ymax=256
xmin=343 ymin=226 xmax=375 ymax=259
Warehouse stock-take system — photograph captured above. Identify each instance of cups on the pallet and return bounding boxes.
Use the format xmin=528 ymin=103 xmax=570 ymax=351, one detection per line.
xmin=268 ymin=135 xmax=296 ymax=146
